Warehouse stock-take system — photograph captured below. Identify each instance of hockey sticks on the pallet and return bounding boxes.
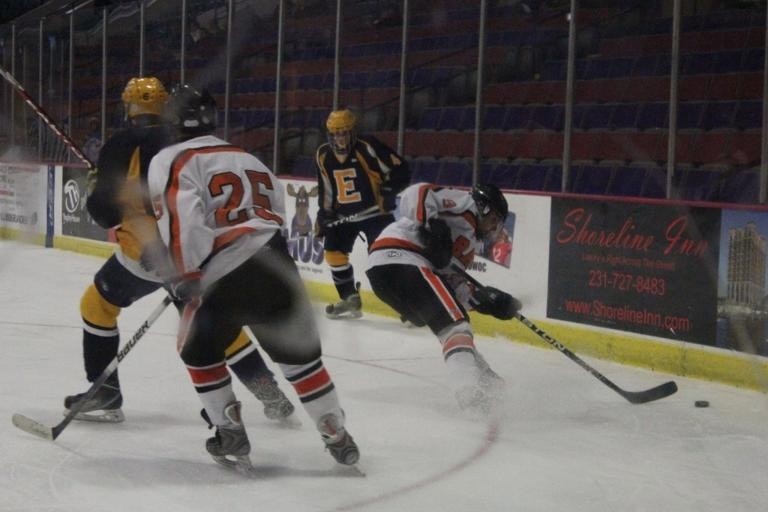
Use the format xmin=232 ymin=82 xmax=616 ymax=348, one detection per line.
xmin=449 ymin=261 xmax=677 ymax=404
xmin=11 ymin=295 xmax=173 ymax=442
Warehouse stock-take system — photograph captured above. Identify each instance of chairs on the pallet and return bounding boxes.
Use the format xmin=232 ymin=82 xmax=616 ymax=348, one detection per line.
xmin=0 ymin=1 xmax=768 ymax=206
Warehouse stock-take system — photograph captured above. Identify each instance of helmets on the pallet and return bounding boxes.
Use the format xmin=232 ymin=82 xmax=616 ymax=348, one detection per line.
xmin=120 ymin=76 xmax=170 ymax=122
xmin=326 ymin=108 xmax=357 ymax=133
xmin=170 ymin=83 xmax=219 ymax=137
xmin=472 ymin=182 xmax=508 ymax=222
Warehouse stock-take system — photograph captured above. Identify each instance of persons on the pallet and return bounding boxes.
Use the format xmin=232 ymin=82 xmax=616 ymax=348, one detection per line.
xmin=367 ymin=180 xmax=518 ymax=411
xmin=141 ymin=81 xmax=361 ymax=466
xmin=314 ymin=107 xmax=416 ymax=320
xmin=64 ymin=75 xmax=295 ymax=420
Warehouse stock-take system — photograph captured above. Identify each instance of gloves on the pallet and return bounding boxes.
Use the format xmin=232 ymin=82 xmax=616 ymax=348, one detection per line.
xmin=86 ymin=168 xmax=98 ymax=197
xmin=313 ymin=214 xmax=339 ymax=239
xmin=418 ymin=219 xmax=453 ymax=270
xmin=139 ymin=243 xmax=202 ymax=304
xmin=376 ymin=186 xmax=397 ymax=212
xmin=469 ymin=286 xmax=522 ymax=321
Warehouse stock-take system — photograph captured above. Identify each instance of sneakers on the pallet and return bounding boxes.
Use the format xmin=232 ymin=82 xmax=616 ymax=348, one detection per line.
xmin=325 ymin=431 xmax=360 ymax=465
xmin=325 ymin=293 xmax=362 ymax=314
xmin=63 ymin=381 xmax=123 ymax=411
xmin=263 ymin=398 xmax=295 ymax=420
xmin=205 ymin=424 xmax=251 ymax=456
